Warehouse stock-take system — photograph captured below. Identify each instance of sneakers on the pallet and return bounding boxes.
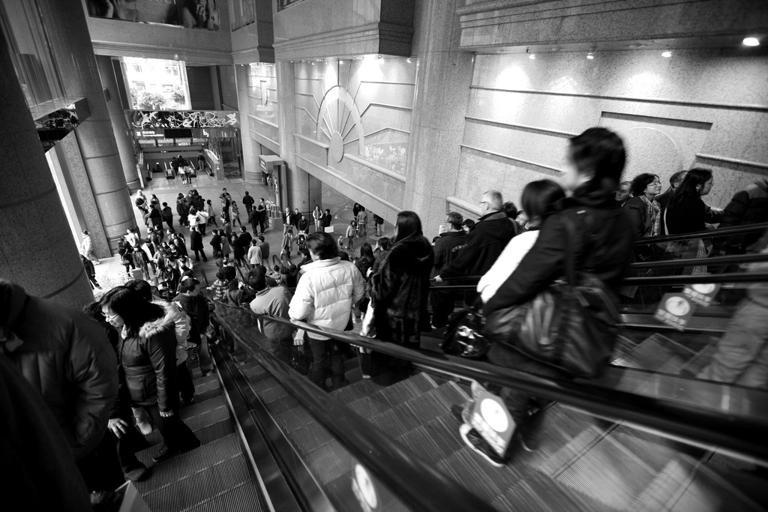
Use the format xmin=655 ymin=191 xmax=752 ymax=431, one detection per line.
xmin=450 ymin=403 xmax=539 ymax=468
xmin=122 ymin=436 xmax=201 ymax=482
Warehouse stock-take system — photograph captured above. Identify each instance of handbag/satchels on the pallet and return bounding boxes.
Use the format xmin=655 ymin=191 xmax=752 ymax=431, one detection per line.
xmin=439 ymin=306 xmax=488 ymax=360
xmin=483 ymin=212 xmax=622 ymax=381
